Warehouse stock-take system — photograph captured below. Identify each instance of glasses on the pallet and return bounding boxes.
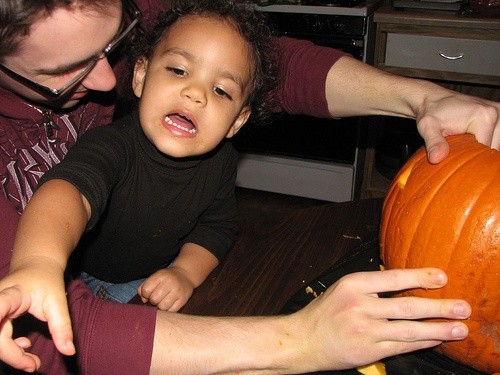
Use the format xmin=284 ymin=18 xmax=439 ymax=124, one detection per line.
xmin=0 ymin=0 xmax=142 ymax=106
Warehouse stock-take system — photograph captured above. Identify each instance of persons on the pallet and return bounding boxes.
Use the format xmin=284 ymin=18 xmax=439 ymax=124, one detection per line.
xmin=0 ymin=0 xmax=285 ymax=375
xmin=0 ymin=0 xmax=500 ymax=374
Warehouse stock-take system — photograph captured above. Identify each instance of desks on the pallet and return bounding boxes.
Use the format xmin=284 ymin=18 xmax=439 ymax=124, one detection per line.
xmin=181 ymin=191 xmax=382 ymax=316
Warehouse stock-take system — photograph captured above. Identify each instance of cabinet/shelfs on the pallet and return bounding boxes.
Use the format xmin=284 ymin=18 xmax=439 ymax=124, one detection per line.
xmin=363 ymin=7 xmax=500 ymax=199
